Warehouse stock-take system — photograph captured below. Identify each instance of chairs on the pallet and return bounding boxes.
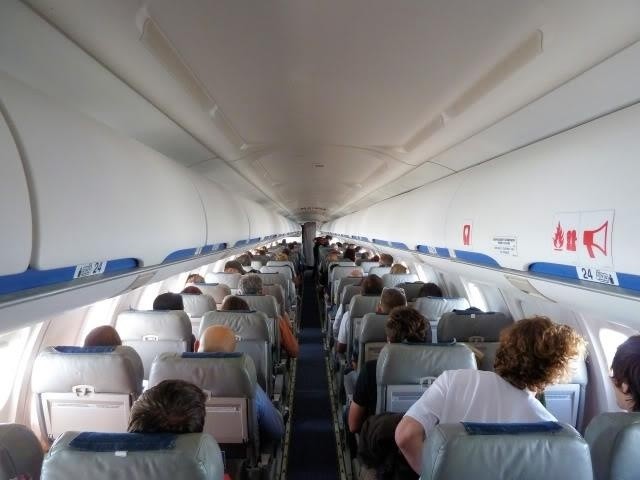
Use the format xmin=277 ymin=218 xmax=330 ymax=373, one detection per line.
xmin=1 ymin=240 xmax=305 ymax=480
xmin=319 ymin=239 xmax=640 ymax=479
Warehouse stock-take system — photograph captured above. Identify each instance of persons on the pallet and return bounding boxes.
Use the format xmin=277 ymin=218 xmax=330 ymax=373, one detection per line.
xmin=125 ymin=376 xmax=231 ymax=480
xmin=360 ymin=316 xmax=588 ymax=479
xmin=84 ymin=235 xmax=484 ymax=452
xmin=609 ymin=335 xmax=640 ymax=412
